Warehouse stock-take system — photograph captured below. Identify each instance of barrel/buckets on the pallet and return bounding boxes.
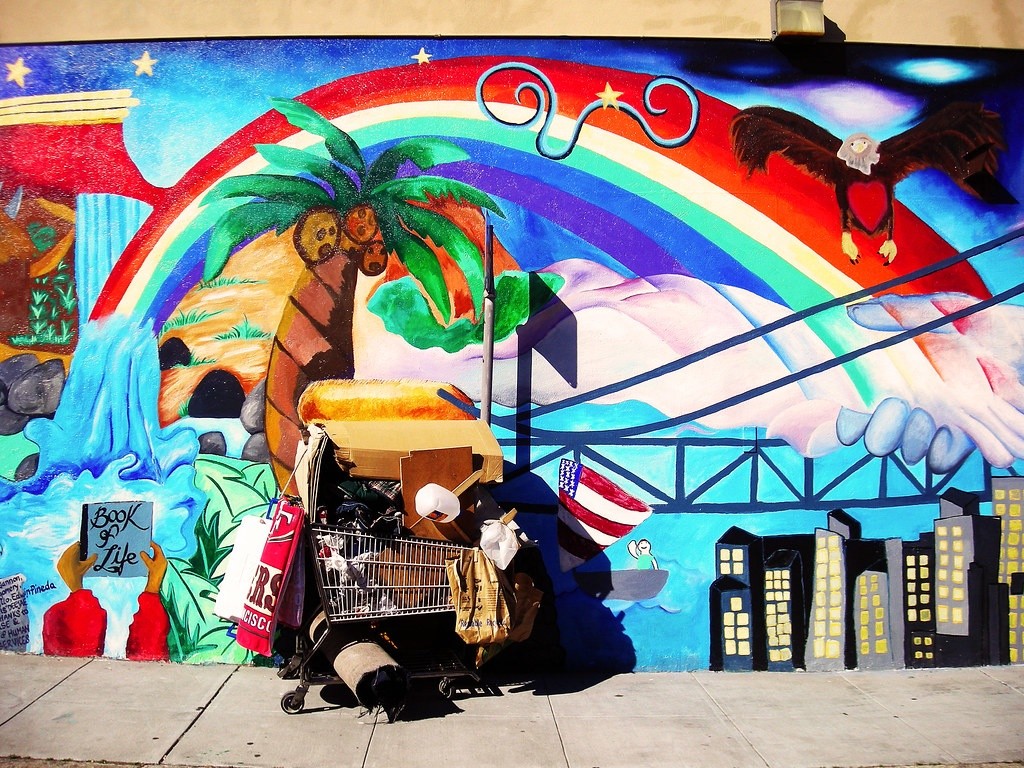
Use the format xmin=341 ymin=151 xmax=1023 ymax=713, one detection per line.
xmin=414 ymin=482 xmax=460 ymax=523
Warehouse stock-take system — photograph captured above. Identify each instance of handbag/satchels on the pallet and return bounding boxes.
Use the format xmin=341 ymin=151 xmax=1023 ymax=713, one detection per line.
xmin=444 ymin=546 xmax=513 ymax=642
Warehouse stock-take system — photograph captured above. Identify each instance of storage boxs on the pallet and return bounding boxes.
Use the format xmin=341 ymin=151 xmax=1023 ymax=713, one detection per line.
xmin=314 ymin=420 xmax=503 ymax=484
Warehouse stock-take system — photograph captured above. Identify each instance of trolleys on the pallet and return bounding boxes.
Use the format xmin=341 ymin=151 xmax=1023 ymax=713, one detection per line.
xmin=277 ymin=505 xmax=505 ymax=714
